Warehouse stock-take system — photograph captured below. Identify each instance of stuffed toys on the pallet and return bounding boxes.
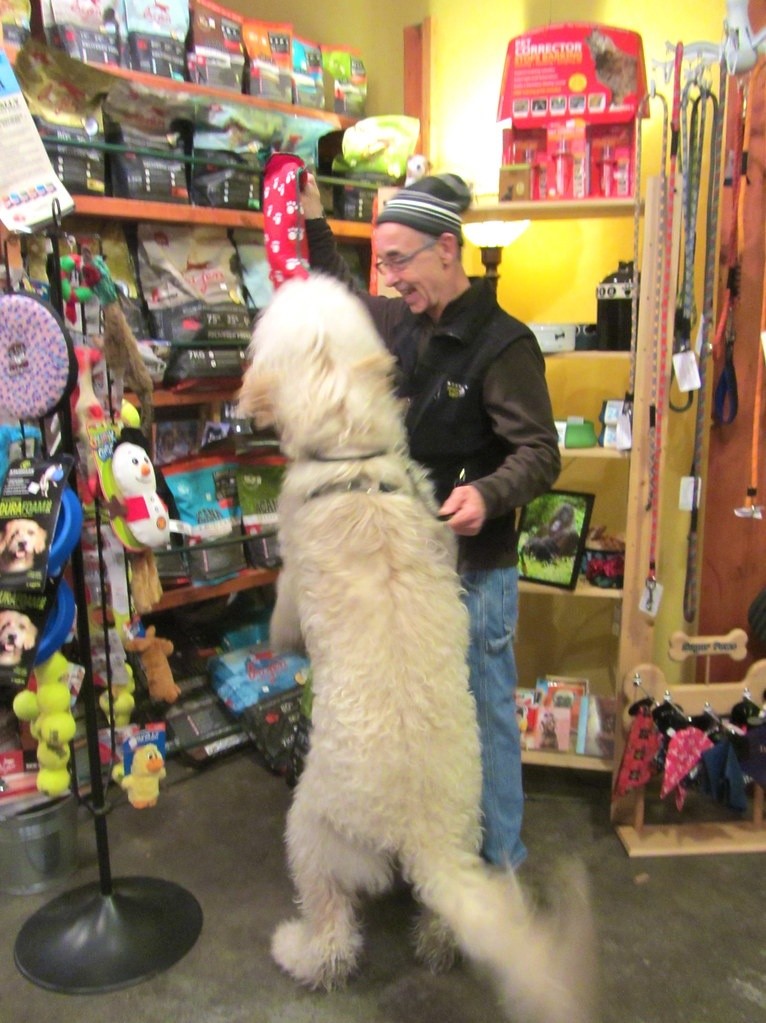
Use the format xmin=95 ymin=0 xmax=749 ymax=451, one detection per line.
xmin=112 ymin=426 xmax=171 ymax=614
xmin=119 ymin=743 xmax=167 ymax=810
xmin=82 ymin=254 xmax=156 ymax=437
xmin=125 ymin=625 xmax=182 ymax=704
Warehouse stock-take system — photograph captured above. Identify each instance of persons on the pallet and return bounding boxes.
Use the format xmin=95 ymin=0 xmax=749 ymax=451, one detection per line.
xmin=299 ymin=172 xmax=562 ymax=985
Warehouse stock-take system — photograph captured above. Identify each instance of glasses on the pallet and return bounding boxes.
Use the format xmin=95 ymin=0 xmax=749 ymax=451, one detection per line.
xmin=375 ymin=241 xmax=436 ymax=276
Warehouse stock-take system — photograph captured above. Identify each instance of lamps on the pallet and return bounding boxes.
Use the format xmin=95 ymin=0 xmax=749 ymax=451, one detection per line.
xmin=458 ymin=219 xmax=532 ymax=293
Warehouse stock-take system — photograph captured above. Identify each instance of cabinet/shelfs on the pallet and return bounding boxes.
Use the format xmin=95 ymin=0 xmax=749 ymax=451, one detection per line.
xmin=0 ymin=41 xmax=401 ymax=776
xmin=448 ymin=199 xmax=642 ymax=772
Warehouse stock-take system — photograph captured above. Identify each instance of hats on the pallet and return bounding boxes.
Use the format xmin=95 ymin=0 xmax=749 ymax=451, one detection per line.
xmin=375 ymin=173 xmax=474 ymax=246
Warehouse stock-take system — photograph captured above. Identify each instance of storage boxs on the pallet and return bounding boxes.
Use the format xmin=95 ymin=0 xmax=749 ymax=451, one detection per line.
xmin=129 ymin=626 xmax=318 ymax=788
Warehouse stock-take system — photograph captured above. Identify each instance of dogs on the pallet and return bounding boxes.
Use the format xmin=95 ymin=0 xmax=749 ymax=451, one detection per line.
xmin=236 ymin=270 xmax=604 ymax=1023
xmin=0 ymin=519 xmax=46 ymax=666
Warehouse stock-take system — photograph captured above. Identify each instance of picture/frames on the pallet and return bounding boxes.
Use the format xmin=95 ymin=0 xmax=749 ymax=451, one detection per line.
xmin=517 ymin=487 xmax=592 ymax=591
